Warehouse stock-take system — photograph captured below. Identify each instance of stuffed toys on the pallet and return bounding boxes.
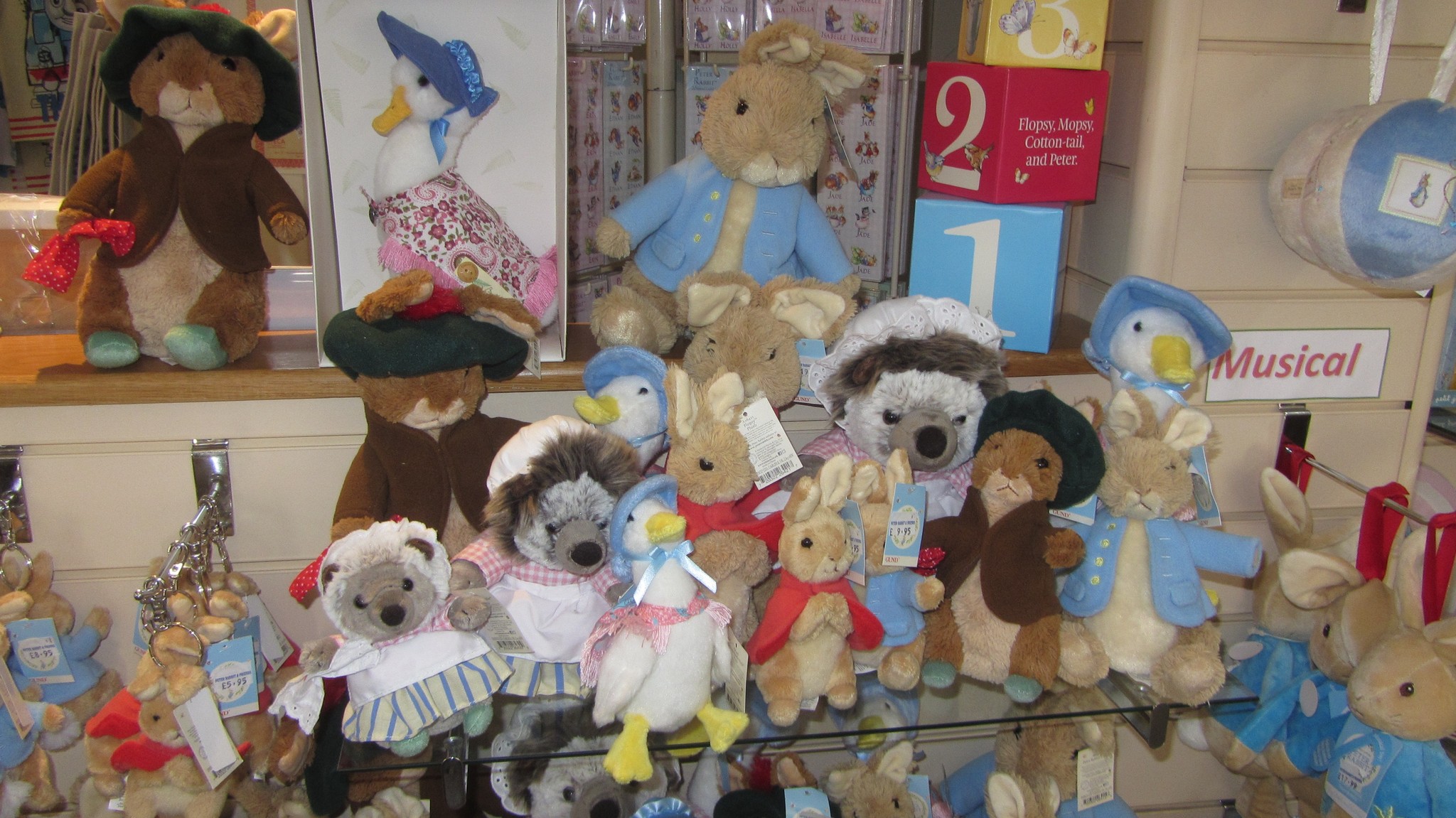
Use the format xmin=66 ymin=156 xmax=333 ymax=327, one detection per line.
xmin=1 ymin=0 xmax=1454 ymax=817
